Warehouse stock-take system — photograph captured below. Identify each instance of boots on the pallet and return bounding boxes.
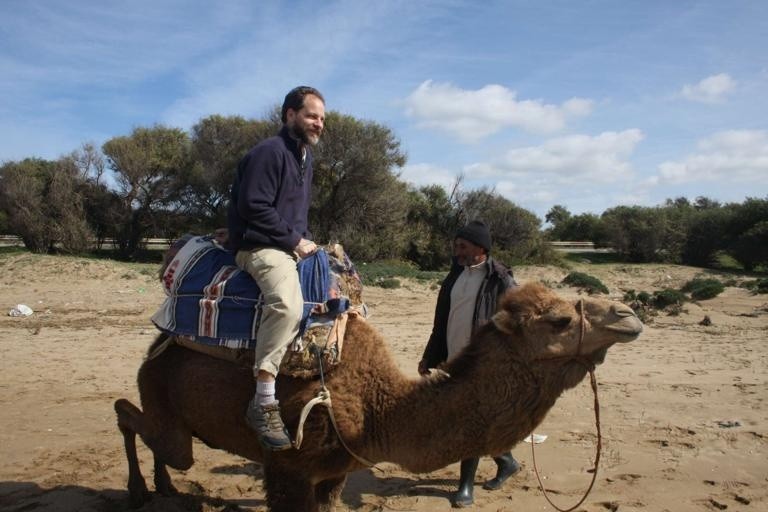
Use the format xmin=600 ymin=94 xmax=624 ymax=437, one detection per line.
xmin=480 ymin=444 xmax=523 ymax=491
xmin=452 ymin=452 xmax=480 ymax=507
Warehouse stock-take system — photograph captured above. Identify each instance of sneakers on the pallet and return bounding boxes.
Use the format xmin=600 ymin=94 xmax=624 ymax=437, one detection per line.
xmin=243 ymin=395 xmax=294 ymax=454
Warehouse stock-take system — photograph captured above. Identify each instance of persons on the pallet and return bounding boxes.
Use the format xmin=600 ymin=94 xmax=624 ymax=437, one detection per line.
xmin=419 ymin=221 xmax=520 ymax=507
xmin=211 ymin=85 xmax=326 ymax=450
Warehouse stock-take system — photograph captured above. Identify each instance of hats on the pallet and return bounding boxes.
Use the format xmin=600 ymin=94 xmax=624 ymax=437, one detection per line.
xmin=452 ymin=219 xmax=493 ymax=252
